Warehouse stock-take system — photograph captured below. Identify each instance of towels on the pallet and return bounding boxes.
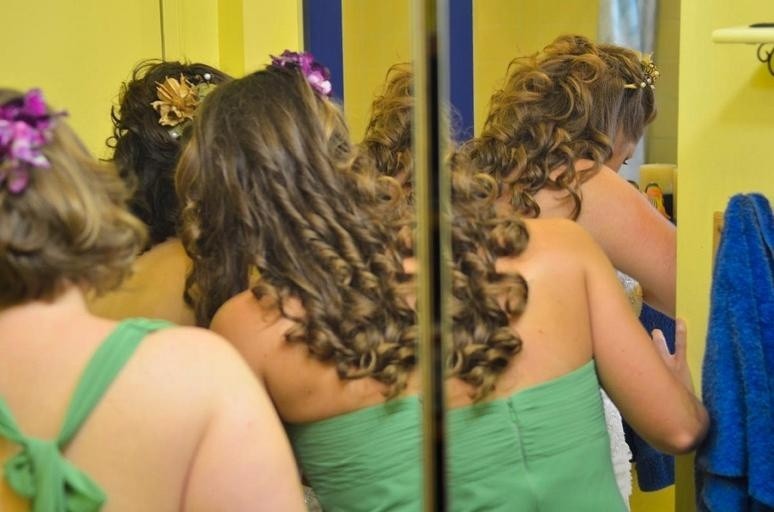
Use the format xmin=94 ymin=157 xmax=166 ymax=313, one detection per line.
xmin=694 ymin=191 xmax=773 ymax=510
xmin=613 ymin=302 xmax=674 ymax=493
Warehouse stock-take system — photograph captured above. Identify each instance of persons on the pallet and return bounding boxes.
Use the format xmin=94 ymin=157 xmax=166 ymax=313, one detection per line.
xmin=0 ymin=83 xmax=314 ymax=512
xmin=76 ymin=52 xmax=241 ymax=332
xmin=168 ymin=61 xmax=430 ymax=511
xmin=440 ymin=148 xmax=713 ymax=511
